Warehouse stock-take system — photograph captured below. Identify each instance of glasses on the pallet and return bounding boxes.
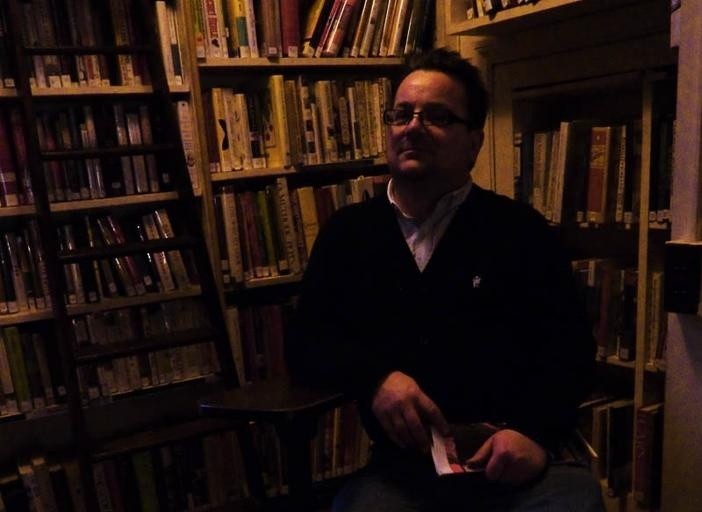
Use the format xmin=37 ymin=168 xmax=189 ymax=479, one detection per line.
xmin=383 ymin=108 xmax=476 ymax=131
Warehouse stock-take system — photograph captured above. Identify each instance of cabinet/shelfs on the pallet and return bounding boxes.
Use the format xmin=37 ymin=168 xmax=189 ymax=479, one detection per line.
xmin=1 ymin=0 xmax=246 ymax=510
xmin=437 ymin=0 xmax=596 ymax=40
xmin=478 ymin=31 xmax=702 ymax=511
xmin=187 ymin=1 xmax=435 ymax=511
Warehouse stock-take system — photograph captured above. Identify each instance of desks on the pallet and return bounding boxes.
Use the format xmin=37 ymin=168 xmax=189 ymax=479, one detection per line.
xmin=197 ymin=380 xmax=359 ymax=510
